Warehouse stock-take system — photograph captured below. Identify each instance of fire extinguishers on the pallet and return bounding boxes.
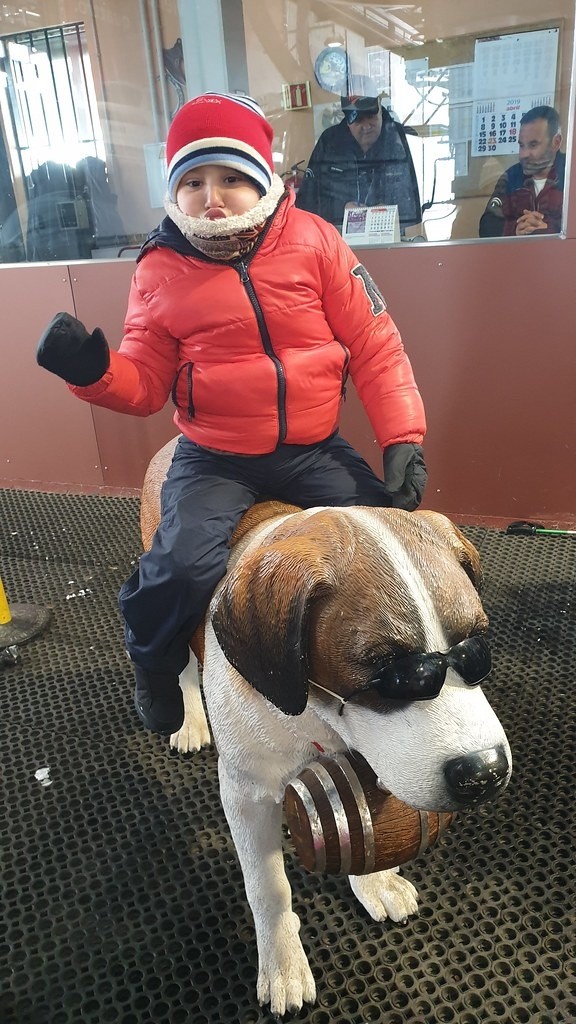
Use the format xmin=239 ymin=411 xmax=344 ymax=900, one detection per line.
xmin=280 ymin=160 xmax=306 ymax=193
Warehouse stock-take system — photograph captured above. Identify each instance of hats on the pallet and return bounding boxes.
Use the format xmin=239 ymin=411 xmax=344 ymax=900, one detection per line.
xmin=167 ymin=91 xmax=275 ymax=205
xmin=339 ymin=75 xmax=379 ymax=125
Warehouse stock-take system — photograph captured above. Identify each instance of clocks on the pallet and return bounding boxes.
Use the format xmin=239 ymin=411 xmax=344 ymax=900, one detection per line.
xmin=313 ymin=46 xmax=351 ymax=91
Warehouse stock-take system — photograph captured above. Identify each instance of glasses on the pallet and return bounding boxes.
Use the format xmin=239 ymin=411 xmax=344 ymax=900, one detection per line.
xmin=335 ymin=634 xmax=493 ymax=716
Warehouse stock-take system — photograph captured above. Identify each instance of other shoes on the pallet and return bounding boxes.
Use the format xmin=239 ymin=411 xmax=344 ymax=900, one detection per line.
xmin=130 ymin=661 xmax=185 ymax=735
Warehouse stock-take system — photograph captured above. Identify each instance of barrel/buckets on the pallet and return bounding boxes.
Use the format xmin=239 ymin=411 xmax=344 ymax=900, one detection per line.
xmin=283 ymin=751 xmax=455 ymax=874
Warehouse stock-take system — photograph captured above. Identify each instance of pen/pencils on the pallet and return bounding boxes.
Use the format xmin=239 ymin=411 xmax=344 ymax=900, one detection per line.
xmin=504 ymin=520 xmax=576 ymax=536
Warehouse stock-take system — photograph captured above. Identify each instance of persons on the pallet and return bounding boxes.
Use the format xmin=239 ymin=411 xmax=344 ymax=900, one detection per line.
xmin=295 ymin=76 xmax=421 ymax=236
xmin=36 ymin=91 xmax=426 ymax=736
xmin=450 ymin=156 xmax=509 ymax=241
xmin=479 ymin=105 xmax=566 ymax=237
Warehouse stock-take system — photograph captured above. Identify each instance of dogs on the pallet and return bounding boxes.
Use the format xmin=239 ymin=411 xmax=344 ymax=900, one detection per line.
xmin=139 ymin=437 xmax=515 ymax=1019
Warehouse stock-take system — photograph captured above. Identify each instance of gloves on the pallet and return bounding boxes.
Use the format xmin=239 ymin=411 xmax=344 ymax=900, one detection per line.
xmin=380 ymin=440 xmax=428 ymax=511
xmin=37 ymin=312 xmax=111 ymax=386
